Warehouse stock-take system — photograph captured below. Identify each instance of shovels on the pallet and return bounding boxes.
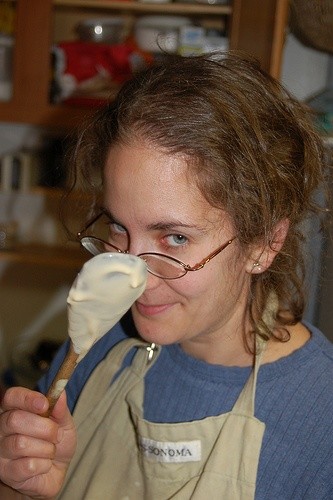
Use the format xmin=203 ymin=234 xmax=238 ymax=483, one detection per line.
xmin=42 ymin=253 xmax=147 ymax=416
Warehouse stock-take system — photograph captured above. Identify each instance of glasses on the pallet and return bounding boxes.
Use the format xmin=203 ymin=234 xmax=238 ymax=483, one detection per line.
xmin=76 ymin=212 xmax=241 ymax=280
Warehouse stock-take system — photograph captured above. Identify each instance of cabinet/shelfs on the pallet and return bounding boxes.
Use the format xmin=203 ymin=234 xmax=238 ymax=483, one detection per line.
xmin=0 ymin=0 xmax=289 ymax=130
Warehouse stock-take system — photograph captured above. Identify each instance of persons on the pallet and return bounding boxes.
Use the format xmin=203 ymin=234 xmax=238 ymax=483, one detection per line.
xmin=0 ymin=32 xmax=333 ymax=500
